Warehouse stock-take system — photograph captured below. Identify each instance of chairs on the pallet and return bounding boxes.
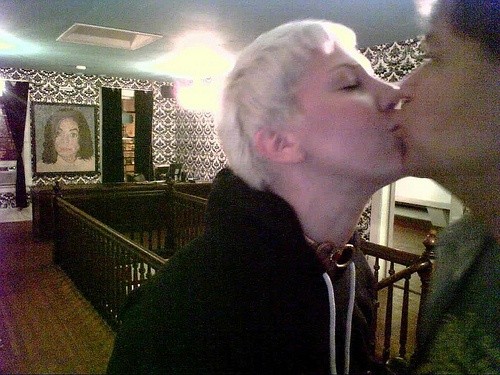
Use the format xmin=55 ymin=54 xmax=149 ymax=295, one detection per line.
xmin=166 ymin=163 xmax=182 ymax=181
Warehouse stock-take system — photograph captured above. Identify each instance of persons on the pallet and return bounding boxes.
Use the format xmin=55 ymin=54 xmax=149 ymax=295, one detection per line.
xmin=36 ymin=108 xmax=95 ymax=172
xmin=104 ymin=18 xmax=404 ymax=375
xmin=404 ymin=0 xmax=500 ymax=375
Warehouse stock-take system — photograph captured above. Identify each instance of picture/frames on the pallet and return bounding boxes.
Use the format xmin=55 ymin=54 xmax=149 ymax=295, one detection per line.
xmin=30 ymin=100 xmax=98 ymax=176
